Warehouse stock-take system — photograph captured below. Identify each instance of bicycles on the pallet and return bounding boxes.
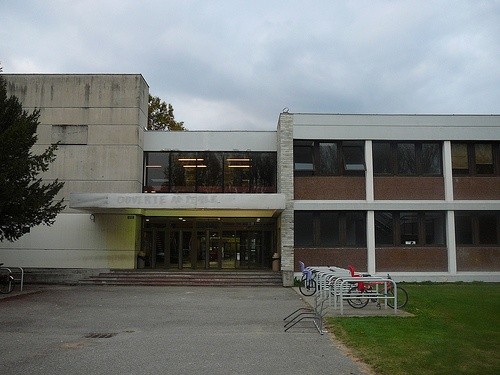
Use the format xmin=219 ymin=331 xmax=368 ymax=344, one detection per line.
xmin=346 ymin=265 xmax=408 ymax=309
xmin=0 ymin=262 xmax=15 ymax=294
xmin=298 ymin=260 xmax=337 ymax=296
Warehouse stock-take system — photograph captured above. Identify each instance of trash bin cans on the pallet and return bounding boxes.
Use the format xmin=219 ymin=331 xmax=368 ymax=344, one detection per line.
xmin=271 ymin=253 xmax=281 ymax=271
